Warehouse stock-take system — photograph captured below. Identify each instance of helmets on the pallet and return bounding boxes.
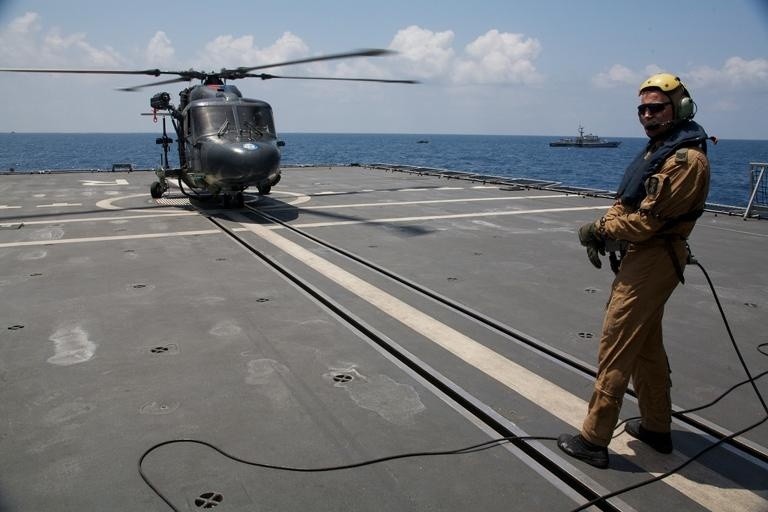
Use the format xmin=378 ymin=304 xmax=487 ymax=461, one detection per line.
xmin=636 ymin=73 xmax=696 ymax=122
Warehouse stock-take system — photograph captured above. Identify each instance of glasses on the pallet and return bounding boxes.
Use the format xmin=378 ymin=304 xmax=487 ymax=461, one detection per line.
xmin=638 ymin=102 xmax=670 ymax=115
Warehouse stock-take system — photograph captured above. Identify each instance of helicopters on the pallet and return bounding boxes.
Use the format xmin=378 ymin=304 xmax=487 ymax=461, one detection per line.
xmin=0 ymin=46 xmax=424 ymax=207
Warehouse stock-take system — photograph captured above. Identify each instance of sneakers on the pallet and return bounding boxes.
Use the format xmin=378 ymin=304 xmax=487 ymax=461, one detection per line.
xmin=557 ymin=432 xmax=610 ymax=469
xmin=624 ymin=419 xmax=674 ymax=455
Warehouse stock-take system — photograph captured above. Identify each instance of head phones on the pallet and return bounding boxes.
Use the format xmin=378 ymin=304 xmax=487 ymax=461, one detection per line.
xmin=679 ymin=85 xmax=698 ymax=120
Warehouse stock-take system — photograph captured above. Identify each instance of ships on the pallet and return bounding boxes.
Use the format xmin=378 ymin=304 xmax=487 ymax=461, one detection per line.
xmin=549 ymin=126 xmax=623 ymax=148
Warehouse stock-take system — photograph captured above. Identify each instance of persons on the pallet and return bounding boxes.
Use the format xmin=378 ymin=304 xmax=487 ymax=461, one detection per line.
xmin=252 ymin=115 xmax=269 ymax=133
xmin=556 ymin=73 xmax=710 ymax=468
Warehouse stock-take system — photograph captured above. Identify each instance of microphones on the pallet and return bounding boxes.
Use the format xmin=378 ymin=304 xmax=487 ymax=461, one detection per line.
xmin=645 ymin=120 xmax=681 ymax=131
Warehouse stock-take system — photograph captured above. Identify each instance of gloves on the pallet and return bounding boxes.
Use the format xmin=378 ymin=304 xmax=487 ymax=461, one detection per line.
xmin=577 ymin=223 xmax=601 ymax=245
xmin=585 ymin=239 xmax=607 ymax=269
